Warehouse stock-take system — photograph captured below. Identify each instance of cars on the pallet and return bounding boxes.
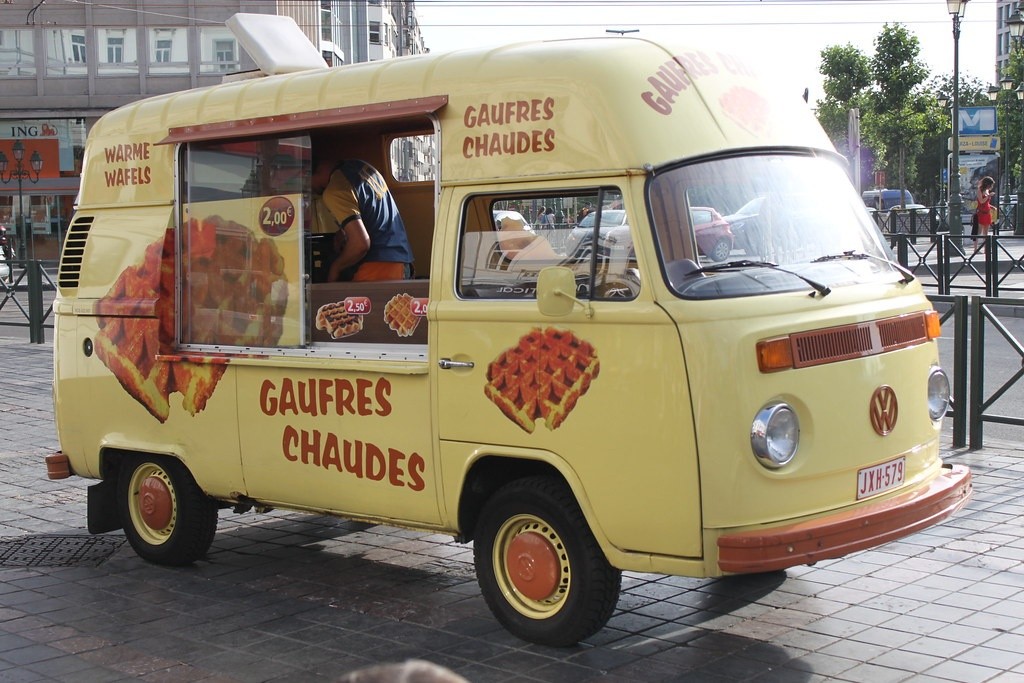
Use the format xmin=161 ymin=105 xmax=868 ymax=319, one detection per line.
xmin=566 ymin=209 xmax=635 ymax=257
xmin=689 ymin=206 xmax=734 ymax=261
xmin=997 ymin=195 xmax=1019 ymax=225
xmin=885 ymin=203 xmax=940 ymax=225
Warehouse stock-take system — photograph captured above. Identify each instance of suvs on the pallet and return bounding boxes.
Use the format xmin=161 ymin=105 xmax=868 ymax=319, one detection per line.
xmin=722 ymin=196 xmax=764 ymax=260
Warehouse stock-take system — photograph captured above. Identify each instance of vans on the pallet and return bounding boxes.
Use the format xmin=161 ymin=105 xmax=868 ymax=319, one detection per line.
xmin=44 ymin=12 xmax=973 ymax=649
xmin=863 ymin=189 xmax=914 ymax=211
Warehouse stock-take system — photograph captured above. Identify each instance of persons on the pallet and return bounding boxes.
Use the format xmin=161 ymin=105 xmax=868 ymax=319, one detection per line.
xmin=538 ymin=206 xmax=556 ymax=229
xmin=974 ymin=176 xmax=996 ymax=254
xmin=312 ymin=157 xmax=417 ymax=281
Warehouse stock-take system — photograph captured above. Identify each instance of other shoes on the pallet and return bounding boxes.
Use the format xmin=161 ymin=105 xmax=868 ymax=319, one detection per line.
xmin=974 ymin=251 xmax=982 ymax=254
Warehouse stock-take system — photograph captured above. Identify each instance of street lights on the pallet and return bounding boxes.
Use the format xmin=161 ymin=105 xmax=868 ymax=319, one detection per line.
xmin=987 ymin=73 xmax=1024 ymax=203
xmin=946 ymin=0 xmax=969 ymax=258
xmin=0 ymin=140 xmax=43 ymax=266
